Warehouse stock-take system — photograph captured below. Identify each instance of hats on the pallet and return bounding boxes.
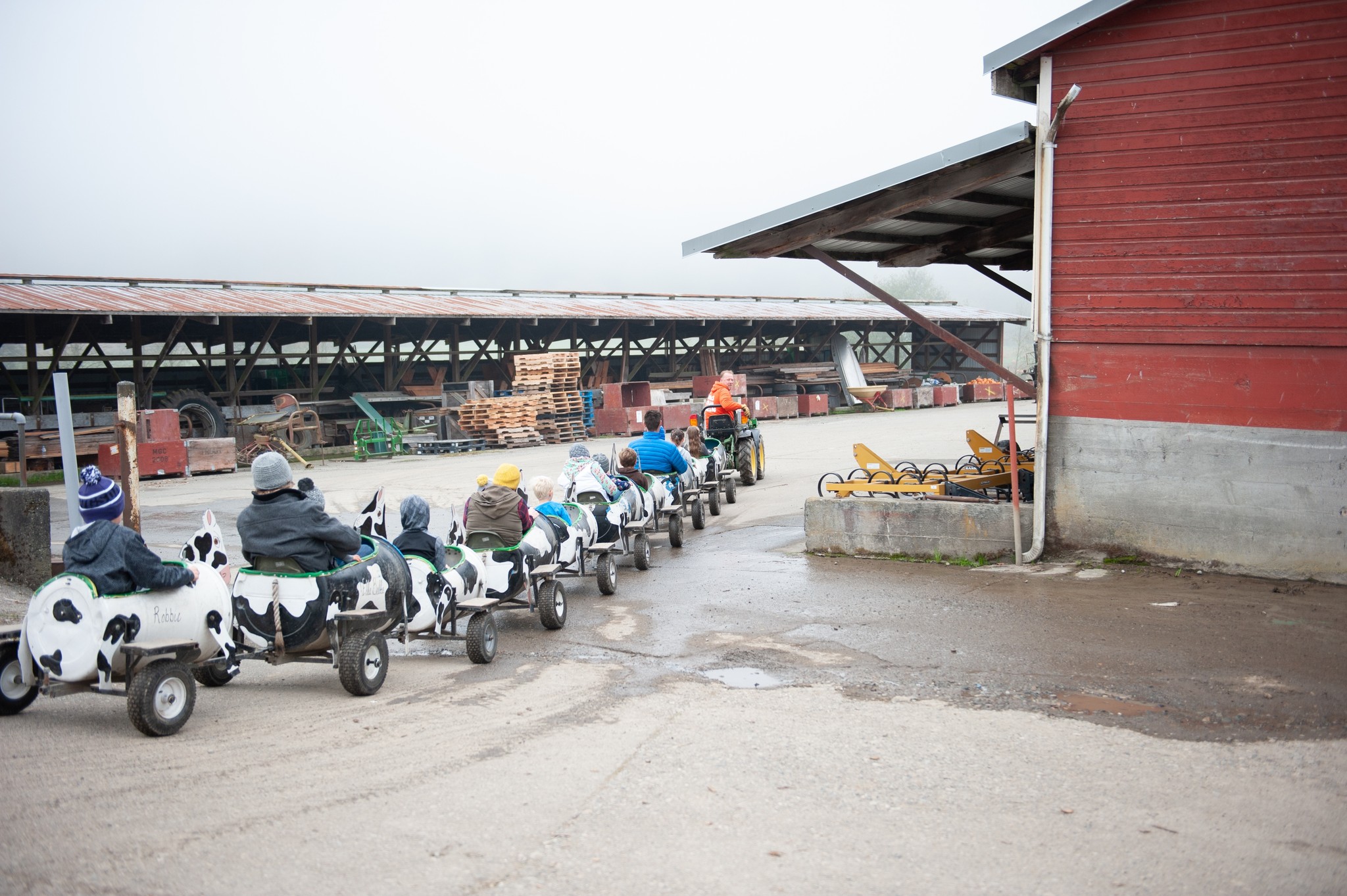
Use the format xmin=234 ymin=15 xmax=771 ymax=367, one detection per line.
xmin=78 ymin=465 xmax=125 ymax=524
xmin=251 ymin=451 xmax=292 ymax=491
xmin=298 ymin=477 xmax=325 ymax=511
xmin=591 ymin=453 xmax=609 ymax=472
xmin=476 ymin=474 xmax=493 ymax=492
xmin=493 ymin=463 xmax=520 ymax=489
xmin=569 ymin=443 xmax=590 ymax=458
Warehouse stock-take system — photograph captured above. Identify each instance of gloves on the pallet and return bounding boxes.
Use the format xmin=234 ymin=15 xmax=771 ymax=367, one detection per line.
xmin=741 ymin=404 xmax=750 ymax=418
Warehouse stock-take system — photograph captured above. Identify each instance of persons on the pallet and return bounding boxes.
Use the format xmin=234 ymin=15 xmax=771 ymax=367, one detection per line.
xmin=62 ymin=464 xmax=200 ymax=595
xmin=686 ymin=426 xmax=719 ymax=456
xmin=557 ymin=443 xmax=624 ymax=503
xmin=705 ymin=369 xmax=751 ymax=429
xmin=463 ymin=462 xmax=540 ymax=547
xmin=617 ymin=447 xmax=649 ymax=491
xmin=670 ymin=429 xmax=696 ymax=468
xmin=391 ymin=494 xmax=452 ymax=573
xmin=533 ymin=475 xmax=572 ymax=527
xmin=591 ymin=452 xmax=633 ymax=502
xmin=628 ymin=410 xmax=687 ymax=474
xmin=236 ymin=451 xmax=373 ymax=573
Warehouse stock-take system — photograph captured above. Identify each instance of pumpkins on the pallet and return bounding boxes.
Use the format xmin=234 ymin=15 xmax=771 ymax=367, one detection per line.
xmin=966 ymin=376 xmax=1000 ymax=385
